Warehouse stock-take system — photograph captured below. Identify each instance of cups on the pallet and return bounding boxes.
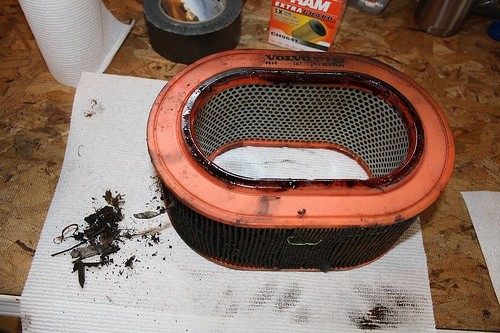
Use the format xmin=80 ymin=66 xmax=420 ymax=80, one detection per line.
xmin=415 ymin=0 xmax=473 ymax=38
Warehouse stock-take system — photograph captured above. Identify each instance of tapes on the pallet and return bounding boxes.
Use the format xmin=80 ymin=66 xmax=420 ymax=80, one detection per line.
xmin=144 ymin=0 xmax=245 ymax=65
